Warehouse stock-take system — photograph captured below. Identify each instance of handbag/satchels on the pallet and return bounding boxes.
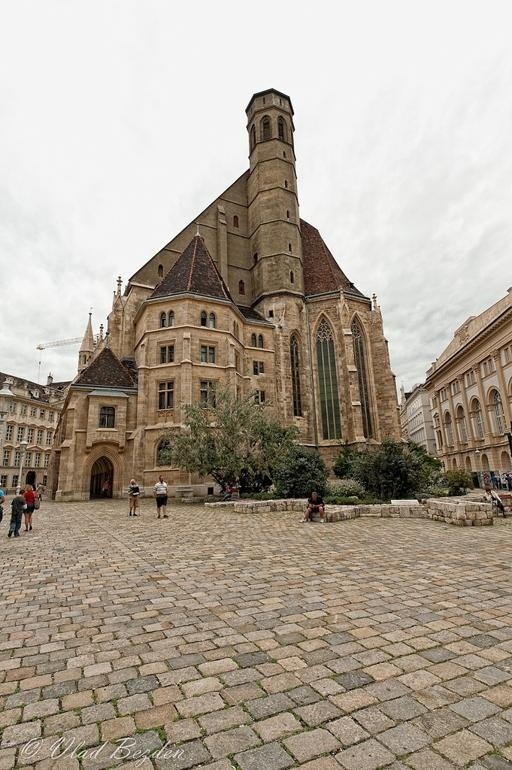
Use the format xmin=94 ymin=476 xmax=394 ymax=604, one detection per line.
xmin=33 ymin=498 xmax=41 ymax=509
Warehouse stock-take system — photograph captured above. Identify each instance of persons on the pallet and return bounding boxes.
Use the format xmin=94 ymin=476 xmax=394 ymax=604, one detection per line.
xmin=102 ymin=480 xmax=110 ymax=498
xmin=299 ymin=491 xmax=326 ymax=523
xmin=153 ymin=474 xmax=170 ymax=519
xmin=483 ymin=487 xmax=507 ymax=519
xmin=481 ymin=471 xmax=512 ymax=492
xmin=0 ymin=479 xmax=45 ymax=539
xmin=127 ymin=478 xmax=141 ymax=517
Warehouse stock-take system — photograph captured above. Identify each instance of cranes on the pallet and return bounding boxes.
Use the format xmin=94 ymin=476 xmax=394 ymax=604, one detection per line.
xmin=35 ymin=333 xmax=100 ymax=350
